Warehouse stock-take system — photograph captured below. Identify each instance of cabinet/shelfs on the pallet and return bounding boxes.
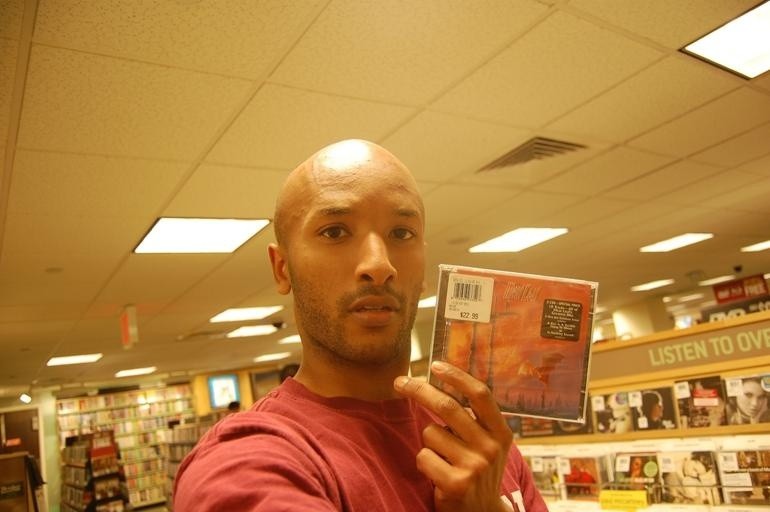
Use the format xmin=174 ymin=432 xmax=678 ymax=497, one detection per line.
xmin=52 ymin=381 xmax=228 ymax=509
xmin=506 ymin=364 xmax=769 ymax=512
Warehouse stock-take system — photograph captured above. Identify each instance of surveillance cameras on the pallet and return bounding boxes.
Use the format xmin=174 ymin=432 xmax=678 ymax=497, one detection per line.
xmin=271 ymin=319 xmax=285 ymax=331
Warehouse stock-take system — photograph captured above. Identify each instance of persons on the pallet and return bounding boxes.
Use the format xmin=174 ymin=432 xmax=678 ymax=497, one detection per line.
xmin=636 ymin=391 xmax=664 ymax=430
xmin=278 ymin=362 xmax=300 ymax=384
xmin=171 ymin=137 xmax=550 ymax=512
xmin=730 ymin=377 xmax=769 ymax=425
xmin=613 ymin=451 xmax=711 ymax=506
xmin=227 ymin=401 xmax=240 ymax=412
